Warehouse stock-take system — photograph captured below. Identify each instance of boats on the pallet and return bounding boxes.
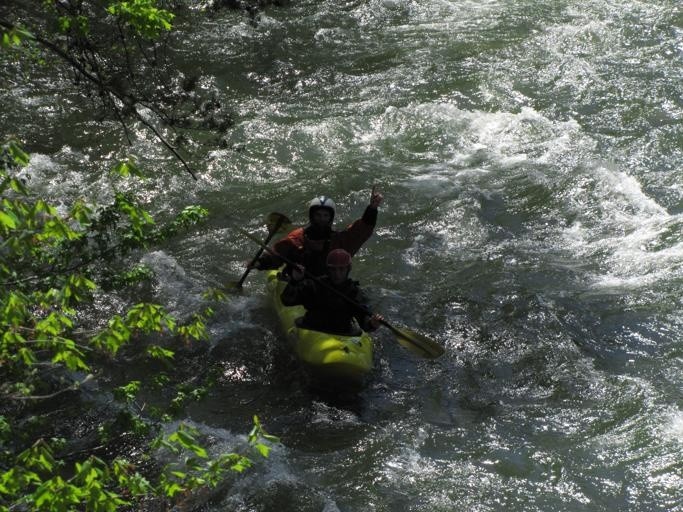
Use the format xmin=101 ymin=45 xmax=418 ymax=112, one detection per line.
xmin=268 ymin=270 xmax=372 ymax=393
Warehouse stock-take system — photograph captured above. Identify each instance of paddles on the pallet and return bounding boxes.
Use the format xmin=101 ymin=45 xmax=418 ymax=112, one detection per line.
xmin=231 ymin=213 xmax=293 ymax=293
xmin=232 ymin=222 xmax=446 ymax=357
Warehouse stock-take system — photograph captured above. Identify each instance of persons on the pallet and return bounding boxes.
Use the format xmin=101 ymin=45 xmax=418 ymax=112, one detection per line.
xmin=277 ymin=247 xmax=389 ymax=337
xmin=243 ymin=184 xmax=382 ymax=285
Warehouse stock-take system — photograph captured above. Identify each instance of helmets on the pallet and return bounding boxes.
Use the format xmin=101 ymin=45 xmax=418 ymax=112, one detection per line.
xmin=326 ymin=248 xmax=352 ymax=271
xmin=308 ymin=196 xmax=336 ymax=224
